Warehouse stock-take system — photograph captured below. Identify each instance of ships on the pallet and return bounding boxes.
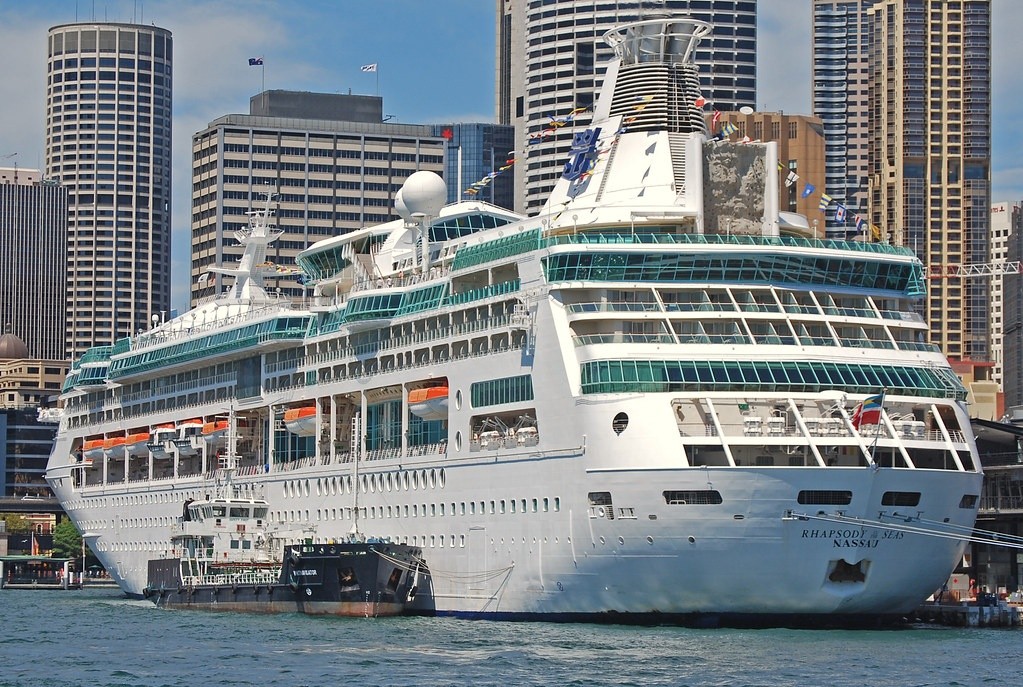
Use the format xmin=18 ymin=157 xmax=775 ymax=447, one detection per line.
xmin=31 ymin=15 xmax=989 ymax=626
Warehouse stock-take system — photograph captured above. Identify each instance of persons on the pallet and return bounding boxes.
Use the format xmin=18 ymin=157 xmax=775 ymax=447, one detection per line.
xmin=97 ymin=570 xmax=108 ymax=579
xmin=89 ymin=570 xmax=92 ymax=575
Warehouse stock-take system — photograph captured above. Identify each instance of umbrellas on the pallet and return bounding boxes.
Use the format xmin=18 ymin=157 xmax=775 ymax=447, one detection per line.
xmin=88 ymin=565 xmax=101 ymax=569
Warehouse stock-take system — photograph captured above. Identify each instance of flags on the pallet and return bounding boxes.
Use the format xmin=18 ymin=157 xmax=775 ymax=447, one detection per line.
xmin=819 ymin=194 xmax=832 ymax=211
xmin=802 ymin=184 xmax=815 ymax=198
xmin=855 ymin=215 xmax=864 ymax=233
xmin=852 ymin=394 xmax=883 ymax=431
xmin=361 ymin=64 xmax=377 ymax=72
xmin=872 ymin=226 xmax=879 ymax=239
xmin=553 ymin=96 xmax=653 ymax=220
xmin=694 ymin=96 xmax=708 ymax=109
xmin=712 ymin=110 xmax=721 ymax=130
xmin=784 ymin=172 xmax=799 ymax=187
xmin=256 ymin=261 xmax=299 ymax=275
xmin=835 ymin=205 xmax=845 ymax=223
xmin=464 ymin=108 xmax=586 ymax=195
xmin=721 ymin=122 xmax=739 ymax=137
xmin=249 ymin=57 xmax=263 ymax=66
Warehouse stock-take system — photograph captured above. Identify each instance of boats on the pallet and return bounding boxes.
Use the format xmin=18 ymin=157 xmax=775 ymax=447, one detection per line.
xmin=83 ymin=439 xmax=104 ymax=463
xmin=173 ymin=417 xmax=203 ymax=455
xmin=283 ymin=405 xmax=320 ymax=438
xmin=146 ymin=423 xmax=174 ymax=460
xmin=142 ymin=404 xmax=437 ymax=621
xmin=102 ymin=437 xmax=125 ymax=460
xmin=124 ymin=432 xmax=149 ymax=458
xmin=408 ymin=385 xmax=449 ymax=422
xmin=199 ymin=420 xmax=228 ymax=448
